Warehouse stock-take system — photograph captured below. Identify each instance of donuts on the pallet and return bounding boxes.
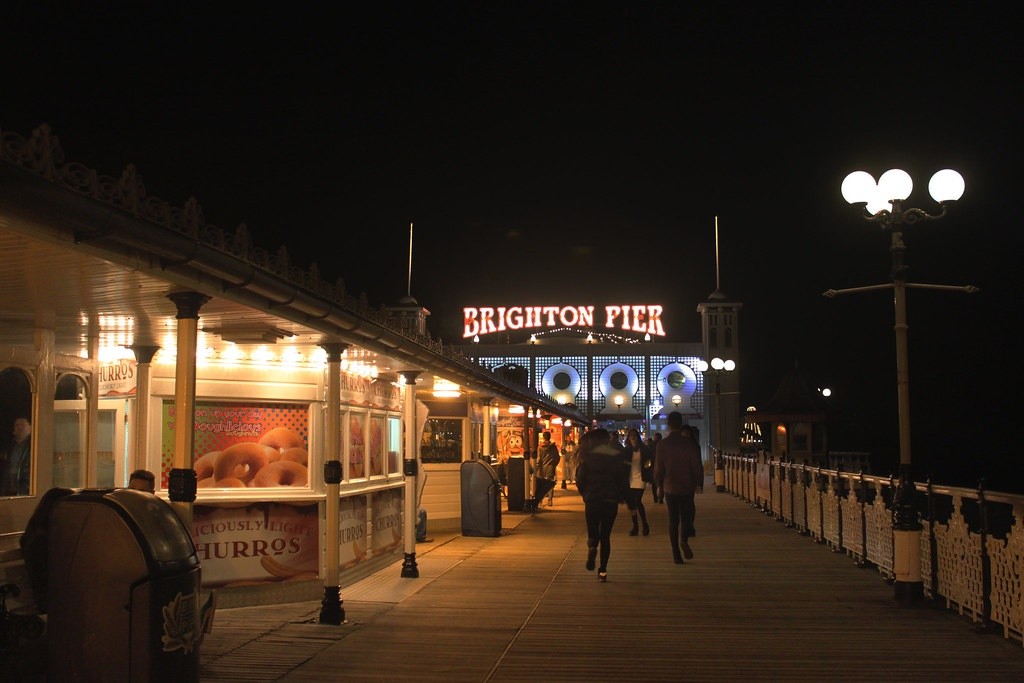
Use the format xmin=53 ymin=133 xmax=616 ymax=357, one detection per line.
xmin=193 ymin=426 xmax=308 ymax=490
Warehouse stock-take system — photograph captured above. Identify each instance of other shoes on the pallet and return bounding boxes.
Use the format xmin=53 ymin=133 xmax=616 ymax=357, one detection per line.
xmin=597 ymin=568 xmax=606 ymax=582
xmin=586 ymin=547 xmax=597 ymax=570
xmin=673 ymin=549 xmax=683 ymax=563
xmin=681 ymin=542 xmax=693 ymax=559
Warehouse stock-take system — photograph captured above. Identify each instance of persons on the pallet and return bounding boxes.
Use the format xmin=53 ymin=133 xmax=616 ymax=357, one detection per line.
xmin=561 ymin=434 xmax=579 ymax=486
xmin=0 ymin=414 xmax=32 ymax=496
xmin=620 ymin=429 xmax=652 ymax=537
xmin=653 ymin=411 xmax=704 ymax=565
xmin=430 ymin=433 xmax=453 ymax=447
xmin=611 ymin=425 xmax=702 ymax=507
xmin=537 ymin=431 xmax=560 ymax=505
xmin=576 ymin=427 xmax=625 ymax=583
xmin=128 ymin=469 xmax=156 ymax=495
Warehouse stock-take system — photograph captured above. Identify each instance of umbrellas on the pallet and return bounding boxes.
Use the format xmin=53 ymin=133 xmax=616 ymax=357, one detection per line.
xmin=535 ymin=464 xmax=556 ymax=506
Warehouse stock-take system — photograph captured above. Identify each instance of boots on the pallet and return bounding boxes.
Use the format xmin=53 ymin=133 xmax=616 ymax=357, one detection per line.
xmin=629 ymin=515 xmax=639 ymax=535
xmin=639 ymin=511 xmax=649 ymax=536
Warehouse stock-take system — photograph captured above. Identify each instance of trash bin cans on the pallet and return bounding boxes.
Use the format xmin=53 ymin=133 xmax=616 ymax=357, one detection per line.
xmin=461 ymin=459 xmax=502 ymax=537
xmin=19 ymin=486 xmax=203 ymax=683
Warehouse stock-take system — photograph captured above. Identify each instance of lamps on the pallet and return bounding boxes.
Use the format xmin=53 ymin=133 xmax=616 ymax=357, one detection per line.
xmin=615 ymin=394 xmax=624 ymax=409
xmin=473 ymin=335 xmax=480 ymax=344
xmin=645 ymin=332 xmax=652 ymax=343
xmin=672 ymin=395 xmax=681 ymax=407
xmin=530 ymin=335 xmax=536 ymax=346
xmin=586 ymin=334 xmax=593 ymax=345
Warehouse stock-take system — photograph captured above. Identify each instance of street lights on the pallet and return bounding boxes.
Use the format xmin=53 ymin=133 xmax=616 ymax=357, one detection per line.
xmin=840 ymin=169 xmax=964 ymax=603
xmin=711 ymin=355 xmax=736 ymax=493
